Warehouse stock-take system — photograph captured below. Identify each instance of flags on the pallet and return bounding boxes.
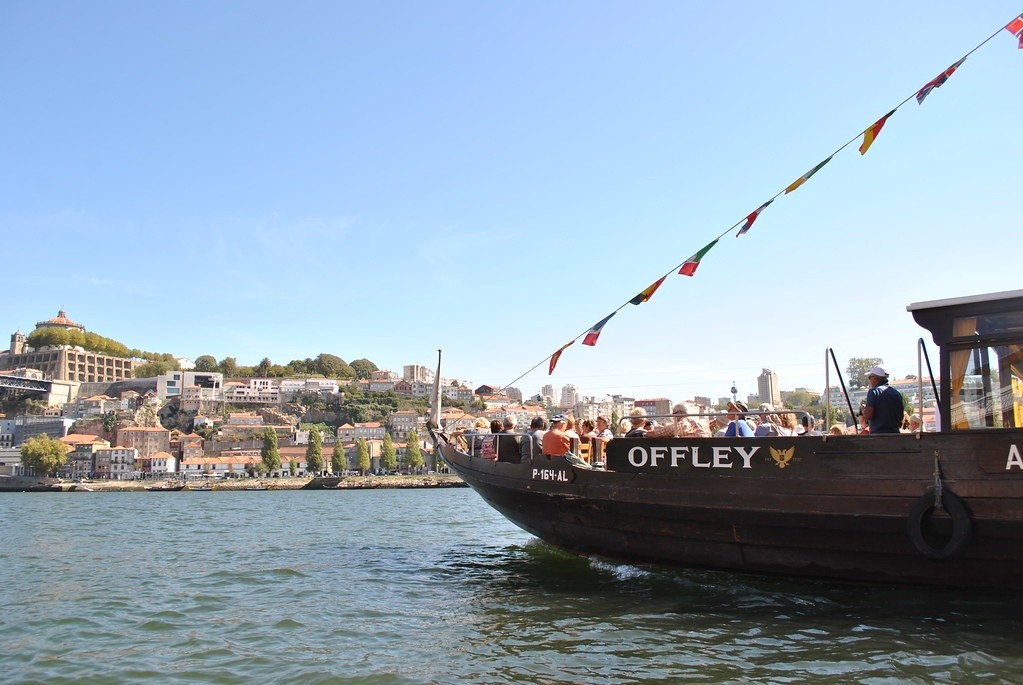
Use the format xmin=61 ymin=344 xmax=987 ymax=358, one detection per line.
xmin=630 ymin=275 xmax=667 ymax=306
xmin=859 ymin=110 xmax=896 ymax=155
xmin=1004 ymin=13 xmax=1023 ymax=49
xmin=549 ymin=341 xmax=575 ymax=375
xmin=736 ymin=200 xmax=774 ymax=237
xmin=581 ymin=312 xmax=615 ymax=346
xmin=916 ymin=59 xmax=966 ymax=105
xmin=678 ymin=239 xmax=719 ymax=277
xmin=785 ymin=156 xmax=833 ymax=194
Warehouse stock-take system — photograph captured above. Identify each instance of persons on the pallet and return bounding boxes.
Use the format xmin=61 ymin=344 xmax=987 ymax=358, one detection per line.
xmin=614 ymin=404 xmax=731 ymax=437
xmin=861 ymin=367 xmax=904 ymax=434
xmin=798 ymin=416 xmax=821 ymax=436
xmin=898 ymin=411 xmax=920 ymax=433
xmin=831 ymin=426 xmax=842 ymax=435
xmin=859 ymin=411 xmax=869 ymax=435
xmin=452 ymin=413 xmax=613 ymax=467
xmin=724 ymin=401 xmax=798 ymax=436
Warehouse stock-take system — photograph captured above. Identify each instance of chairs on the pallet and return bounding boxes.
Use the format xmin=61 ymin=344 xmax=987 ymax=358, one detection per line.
xmin=579 ymin=441 xmax=606 ymax=465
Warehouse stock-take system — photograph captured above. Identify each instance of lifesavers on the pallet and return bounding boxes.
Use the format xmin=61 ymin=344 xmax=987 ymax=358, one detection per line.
xmin=906 ymin=489 xmax=974 ymax=564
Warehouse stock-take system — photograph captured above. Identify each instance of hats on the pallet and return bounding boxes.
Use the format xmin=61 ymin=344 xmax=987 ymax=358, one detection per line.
xmin=550 ymin=414 xmax=568 ymax=423
xmin=727 ymin=401 xmax=748 ymax=412
xmin=864 ymin=367 xmax=889 ymax=377
xmin=802 ymin=415 xmax=814 ymax=424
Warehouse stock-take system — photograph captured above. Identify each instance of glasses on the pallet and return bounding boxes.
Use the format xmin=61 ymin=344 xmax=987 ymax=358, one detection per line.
xmin=868 ymin=376 xmax=876 ymax=379
